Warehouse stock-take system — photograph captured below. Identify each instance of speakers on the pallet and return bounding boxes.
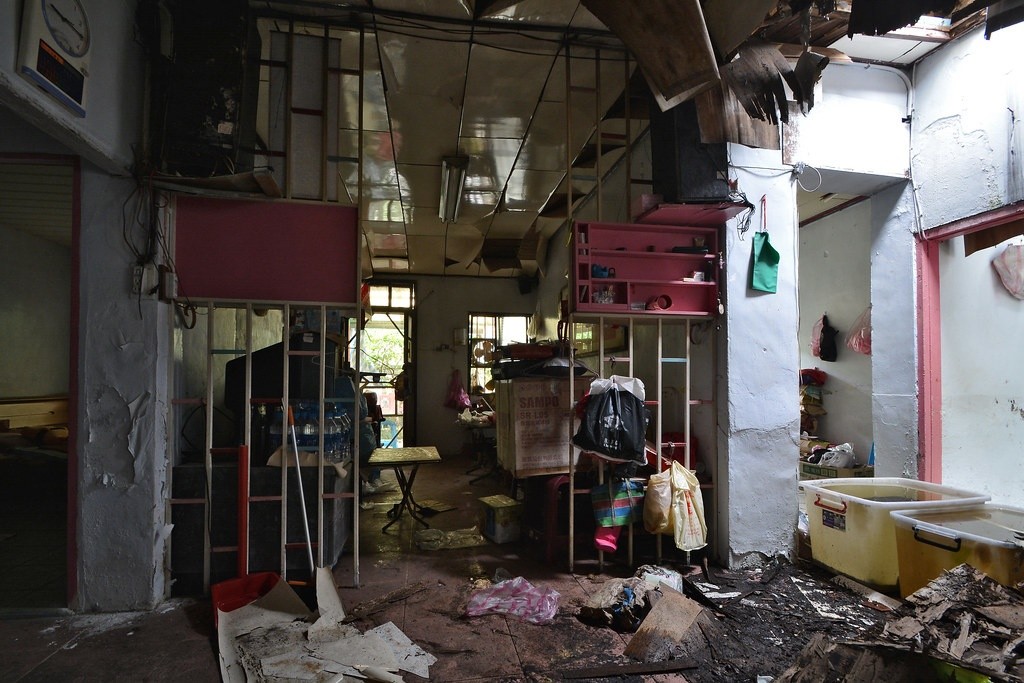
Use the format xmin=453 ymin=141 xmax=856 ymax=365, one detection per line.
xmin=651 ymin=44 xmax=729 ymax=203
xmin=160 ymin=0 xmax=261 ymax=178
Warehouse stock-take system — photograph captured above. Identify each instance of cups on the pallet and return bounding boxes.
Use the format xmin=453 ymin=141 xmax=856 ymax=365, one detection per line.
xmin=695 ymin=237 xmax=704 ymax=246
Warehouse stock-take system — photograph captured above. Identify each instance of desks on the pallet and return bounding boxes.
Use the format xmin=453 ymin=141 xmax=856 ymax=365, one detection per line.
xmin=368 ymin=446 xmax=442 ymax=529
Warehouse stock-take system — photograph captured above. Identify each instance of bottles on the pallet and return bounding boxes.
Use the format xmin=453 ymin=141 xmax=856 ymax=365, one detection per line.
xmin=288 ymin=412 xmax=318 ymax=446
xmin=325 ymin=409 xmax=351 ymax=463
xmin=270 ymin=412 xmax=283 ymax=450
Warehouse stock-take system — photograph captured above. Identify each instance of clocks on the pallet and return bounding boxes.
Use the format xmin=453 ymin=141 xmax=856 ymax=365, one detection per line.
xmin=17 ymin=0 xmax=94 ymax=118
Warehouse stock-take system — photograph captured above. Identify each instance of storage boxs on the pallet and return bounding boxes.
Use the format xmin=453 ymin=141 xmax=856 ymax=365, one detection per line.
xmin=799 ymin=476 xmax=1023 ymax=608
xmin=494 ymin=375 xmax=602 ymax=476
xmin=478 ymin=494 xmax=524 ymax=545
